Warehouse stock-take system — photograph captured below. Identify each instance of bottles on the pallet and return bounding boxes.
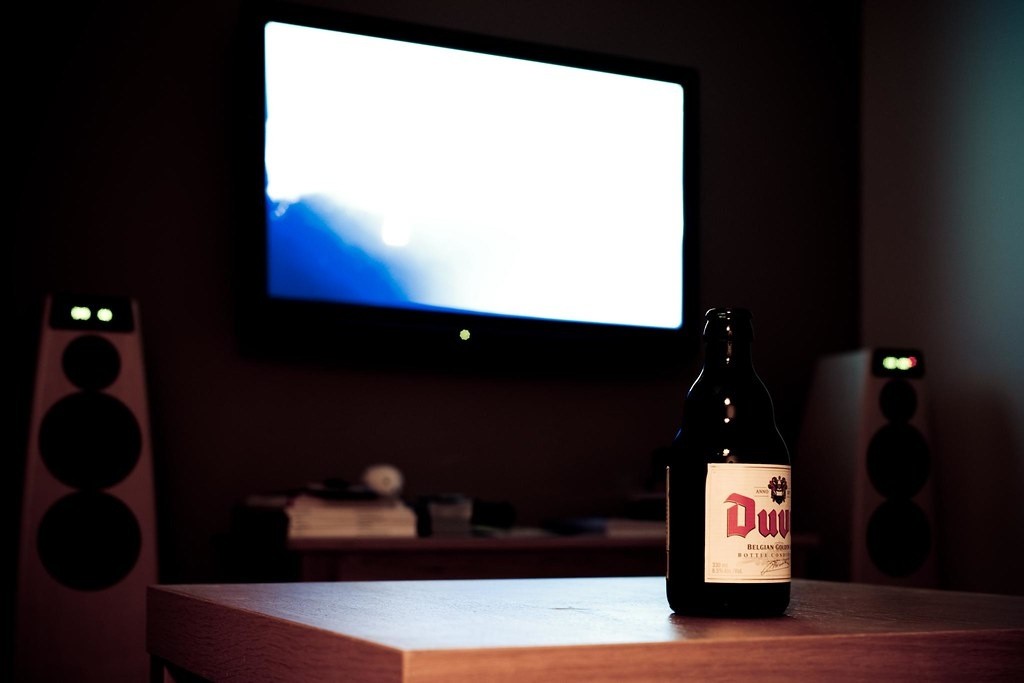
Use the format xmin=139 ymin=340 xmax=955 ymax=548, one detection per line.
xmin=666 ymin=308 xmax=792 ymax=619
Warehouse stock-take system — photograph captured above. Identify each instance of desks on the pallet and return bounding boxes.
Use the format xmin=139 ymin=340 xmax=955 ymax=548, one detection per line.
xmin=249 ymin=495 xmax=666 ymax=582
xmin=146 ymin=577 xmax=1024 ymax=683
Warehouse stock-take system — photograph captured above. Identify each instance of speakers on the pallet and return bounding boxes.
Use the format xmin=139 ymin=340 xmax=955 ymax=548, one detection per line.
xmin=796 ymin=347 xmax=935 ymax=588
xmin=11 ymin=289 xmax=155 ymax=683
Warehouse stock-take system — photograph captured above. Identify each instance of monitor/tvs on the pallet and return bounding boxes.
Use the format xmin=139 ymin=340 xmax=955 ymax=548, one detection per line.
xmin=227 ymin=1 xmax=708 ymax=387
